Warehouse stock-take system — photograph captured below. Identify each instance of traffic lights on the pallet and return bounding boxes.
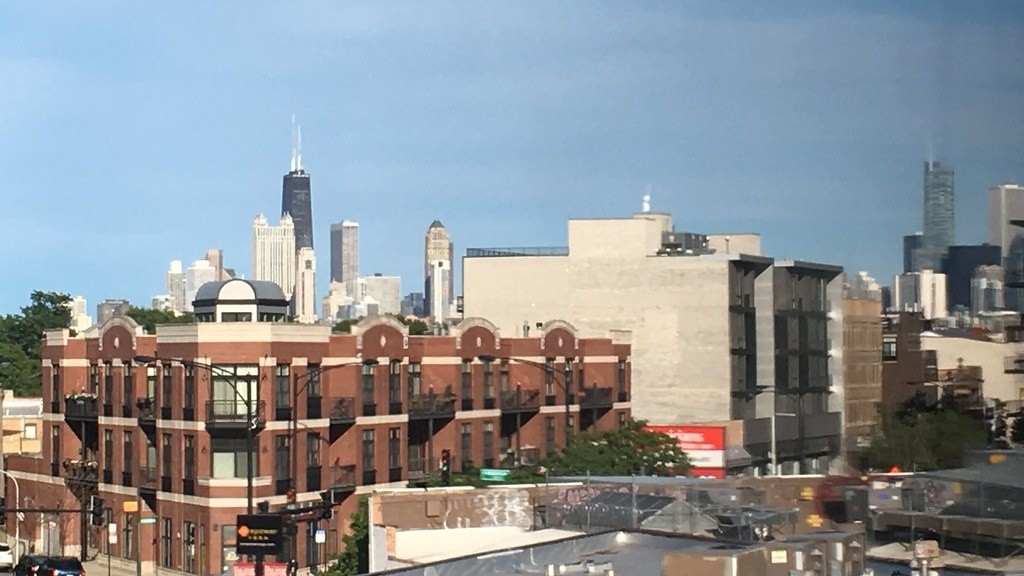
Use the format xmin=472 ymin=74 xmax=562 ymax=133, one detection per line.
xmin=0 ymin=497 xmax=8 ymax=526
xmin=441 ymin=449 xmax=451 ymax=483
xmin=89 ymin=496 xmax=107 ymax=527
xmin=319 ymin=501 xmax=334 ymax=520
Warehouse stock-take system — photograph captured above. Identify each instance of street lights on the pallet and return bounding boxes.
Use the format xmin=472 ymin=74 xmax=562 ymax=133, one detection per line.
xmin=288 ymin=359 xmax=379 ymax=564
xmin=134 ymin=354 xmax=255 ymax=516
xmin=770 ymin=413 xmax=796 ymax=478
xmin=478 ymin=353 xmax=571 ymax=450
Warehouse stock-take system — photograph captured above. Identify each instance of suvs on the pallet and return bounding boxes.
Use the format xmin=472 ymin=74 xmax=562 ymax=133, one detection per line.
xmin=14 ymin=555 xmax=51 ymax=576
xmin=0 ymin=543 xmax=14 ymax=573
xmin=35 ymin=556 xmax=86 ymax=576
